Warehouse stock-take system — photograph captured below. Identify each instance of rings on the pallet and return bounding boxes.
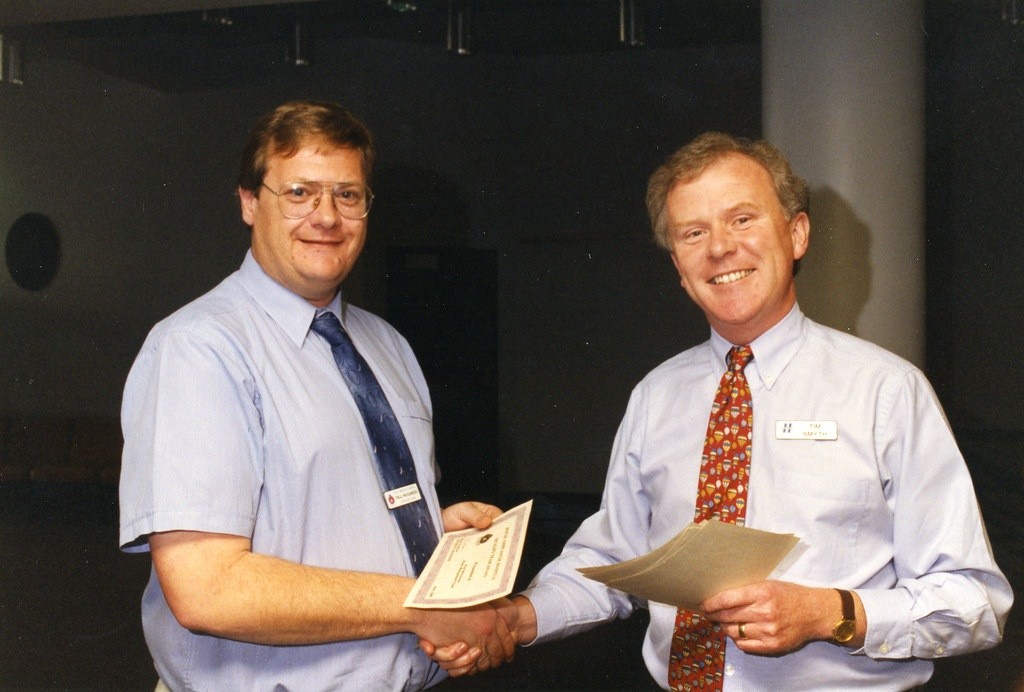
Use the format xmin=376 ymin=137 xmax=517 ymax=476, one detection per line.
xmin=739 ymin=623 xmax=748 ymax=640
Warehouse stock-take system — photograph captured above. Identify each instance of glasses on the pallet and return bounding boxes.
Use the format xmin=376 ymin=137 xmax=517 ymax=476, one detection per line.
xmin=260 ymin=181 xmax=375 ymax=219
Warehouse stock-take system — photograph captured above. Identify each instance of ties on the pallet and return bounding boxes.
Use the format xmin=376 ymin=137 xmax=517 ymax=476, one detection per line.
xmin=310 ymin=312 xmax=440 ymax=579
xmin=668 ymin=345 xmax=754 ymax=692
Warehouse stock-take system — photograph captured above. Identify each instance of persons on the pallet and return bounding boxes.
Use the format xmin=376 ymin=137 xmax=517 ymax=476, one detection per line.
xmin=419 ymin=132 xmax=1014 ymax=692
xmin=120 ymin=102 xmax=515 ymax=692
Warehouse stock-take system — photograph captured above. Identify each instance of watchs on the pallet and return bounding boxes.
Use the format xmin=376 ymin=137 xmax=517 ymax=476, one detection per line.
xmin=829 ymin=589 xmax=855 ymax=647
